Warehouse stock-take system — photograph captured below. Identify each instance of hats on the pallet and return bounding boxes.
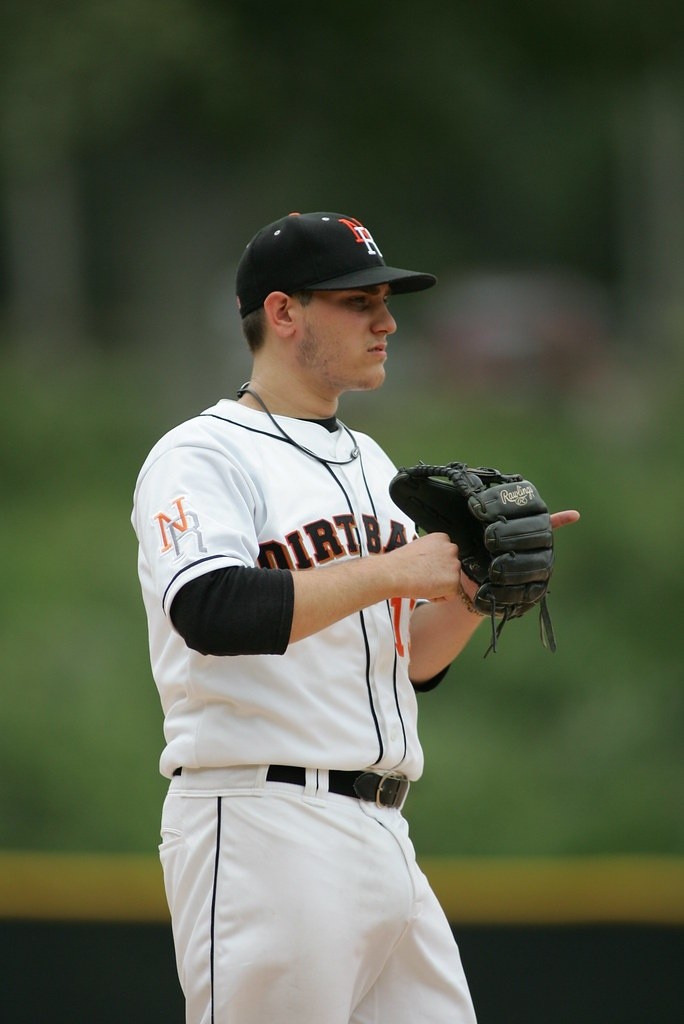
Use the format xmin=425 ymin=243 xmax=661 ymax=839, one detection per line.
xmin=234 ymin=211 xmax=438 ymax=318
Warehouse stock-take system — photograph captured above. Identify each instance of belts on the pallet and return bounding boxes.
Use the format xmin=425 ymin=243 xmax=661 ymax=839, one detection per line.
xmin=173 ymin=765 xmax=409 ymax=813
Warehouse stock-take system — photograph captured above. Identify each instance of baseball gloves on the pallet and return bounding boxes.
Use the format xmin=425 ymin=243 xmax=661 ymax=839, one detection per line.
xmin=390 ymin=460 xmax=559 ymax=662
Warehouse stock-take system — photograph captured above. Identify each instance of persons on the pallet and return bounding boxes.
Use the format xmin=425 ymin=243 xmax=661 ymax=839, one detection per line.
xmin=129 ymin=207 xmax=582 ymax=1023
